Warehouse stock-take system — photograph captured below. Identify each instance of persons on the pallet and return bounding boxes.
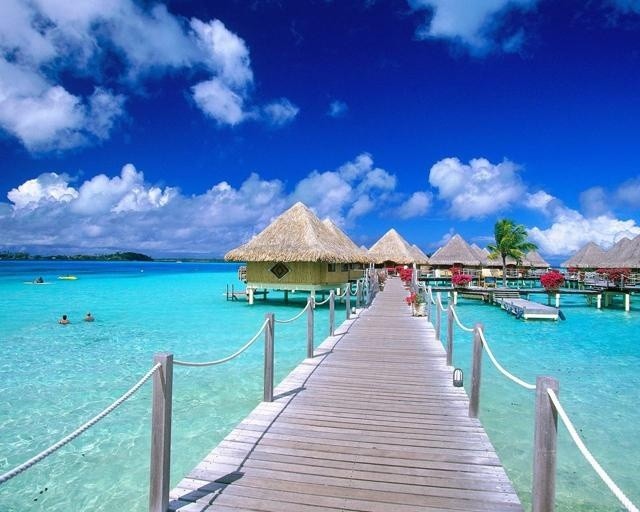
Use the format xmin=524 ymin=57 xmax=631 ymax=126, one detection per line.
xmin=85 ymin=311 xmax=92 ymax=321
xmin=58 ymin=315 xmax=69 ymax=324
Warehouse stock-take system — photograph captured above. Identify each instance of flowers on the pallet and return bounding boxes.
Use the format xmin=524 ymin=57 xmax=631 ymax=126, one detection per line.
xmin=406 ymin=292 xmax=425 ymax=302
xmin=539 ymin=270 xmax=565 ymax=292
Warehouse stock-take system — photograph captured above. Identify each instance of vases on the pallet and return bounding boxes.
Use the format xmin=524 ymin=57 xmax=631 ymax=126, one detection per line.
xmin=548 ymin=293 xmax=560 ymax=308
xmin=413 ymin=303 xmax=426 ymax=317
